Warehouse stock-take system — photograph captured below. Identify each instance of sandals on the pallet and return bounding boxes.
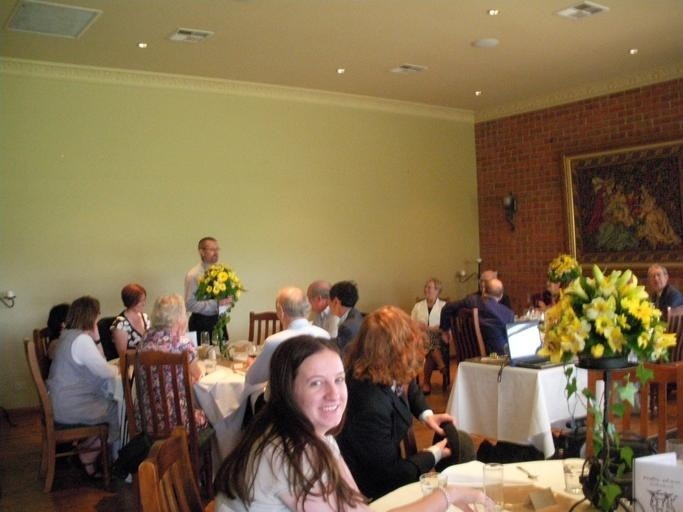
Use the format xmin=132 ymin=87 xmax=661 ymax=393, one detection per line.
xmin=71 ymin=454 xmax=101 ymax=480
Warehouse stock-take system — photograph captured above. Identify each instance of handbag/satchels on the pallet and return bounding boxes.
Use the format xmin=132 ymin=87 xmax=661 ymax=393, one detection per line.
xmin=110 ymin=432 xmax=151 ymax=480
xmin=590 ymin=431 xmax=658 ymax=499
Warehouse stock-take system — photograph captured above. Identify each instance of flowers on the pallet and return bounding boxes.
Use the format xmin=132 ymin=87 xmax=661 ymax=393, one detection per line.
xmin=193 ymin=262 xmax=247 ymax=344
xmin=537 ymin=253 xmax=678 ymax=506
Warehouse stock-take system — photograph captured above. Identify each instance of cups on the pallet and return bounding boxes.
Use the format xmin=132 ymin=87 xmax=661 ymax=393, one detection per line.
xmin=200 ymin=330 xmax=211 ymax=351
xmin=211 ymin=331 xmax=219 ymax=346
xmin=418 ymin=471 xmax=449 ymax=499
xmin=563 ymin=457 xmax=590 ymax=495
xmin=516 ymin=306 xmax=544 ymax=322
xmin=246 ymin=343 xmax=257 ymax=359
xmin=481 ymin=460 xmax=504 ymax=512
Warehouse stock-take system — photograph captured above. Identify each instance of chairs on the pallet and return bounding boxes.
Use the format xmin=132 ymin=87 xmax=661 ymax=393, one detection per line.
xmin=24 ymin=297 xmax=683 ymax=512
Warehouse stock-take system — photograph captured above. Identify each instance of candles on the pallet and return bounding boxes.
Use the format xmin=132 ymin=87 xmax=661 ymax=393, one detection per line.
xmin=5 ymin=288 xmax=15 ymax=297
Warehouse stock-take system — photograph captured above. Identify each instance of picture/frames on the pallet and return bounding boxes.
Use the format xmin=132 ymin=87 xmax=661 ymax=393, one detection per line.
xmin=560 ymin=134 xmax=683 ymax=275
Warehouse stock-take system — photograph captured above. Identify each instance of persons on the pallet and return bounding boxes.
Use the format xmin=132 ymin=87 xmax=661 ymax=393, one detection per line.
xmin=46 ymin=303 xmax=70 ymax=447
xmin=529 ymin=281 xmax=561 ymax=311
xmin=240 ymin=287 xmax=331 ymax=432
xmin=332 ymin=305 xmax=457 ymax=505
xmin=462 ymin=279 xmax=515 ymax=356
xmin=409 ymin=279 xmax=447 ymax=394
xmin=48 ymin=296 xmax=130 ymax=482
xmin=112 ymin=284 xmax=151 ymax=356
xmin=434 ymin=486 xmax=450 ymax=510
xmin=437 ymin=271 xmax=511 ymax=342
xmin=305 ymin=279 xmax=338 ymax=338
xmin=330 ymin=281 xmax=365 ymax=349
xmin=134 ymin=293 xmax=216 ymax=451
xmin=182 ymin=237 xmax=233 ymax=345
xmin=646 ymin=264 xmax=681 ymax=397
xmin=212 ymin=334 xmax=493 ymax=512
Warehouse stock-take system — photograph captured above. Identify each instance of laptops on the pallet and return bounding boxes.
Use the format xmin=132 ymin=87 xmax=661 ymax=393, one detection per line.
xmin=505 ymin=321 xmax=564 ymax=369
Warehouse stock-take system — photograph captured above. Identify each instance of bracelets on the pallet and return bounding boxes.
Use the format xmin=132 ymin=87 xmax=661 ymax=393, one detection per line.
xmin=93 ymin=340 xmax=101 ymax=344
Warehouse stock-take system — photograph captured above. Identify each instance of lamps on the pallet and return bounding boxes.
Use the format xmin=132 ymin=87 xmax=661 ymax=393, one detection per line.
xmin=502 ymin=191 xmax=517 ymax=234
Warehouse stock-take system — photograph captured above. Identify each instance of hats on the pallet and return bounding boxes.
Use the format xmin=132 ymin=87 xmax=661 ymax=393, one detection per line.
xmin=432 ymin=421 xmax=475 ymax=470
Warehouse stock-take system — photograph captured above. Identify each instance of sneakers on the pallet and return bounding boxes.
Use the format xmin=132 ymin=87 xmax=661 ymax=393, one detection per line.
xmin=423 ymin=384 xmax=432 ymax=395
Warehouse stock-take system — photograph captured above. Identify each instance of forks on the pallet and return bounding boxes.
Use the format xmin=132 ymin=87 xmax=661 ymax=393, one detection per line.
xmin=514 ymin=462 xmax=541 ymax=481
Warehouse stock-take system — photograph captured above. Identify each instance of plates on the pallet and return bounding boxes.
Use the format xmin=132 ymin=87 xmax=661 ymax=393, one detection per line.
xmin=438 ymin=465 xmax=496 ymax=489
